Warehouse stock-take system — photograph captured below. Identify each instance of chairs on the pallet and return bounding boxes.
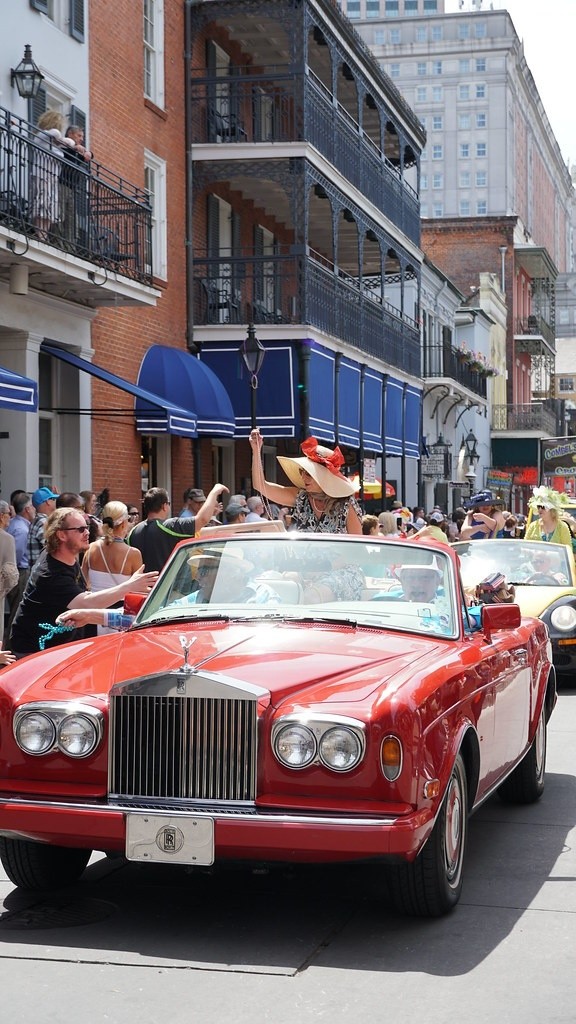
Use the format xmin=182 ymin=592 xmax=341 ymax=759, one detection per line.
xmin=91 ymin=225 xmax=138 ymax=274
xmin=0 ymin=190 xmax=29 ymax=229
xmin=252 ymin=303 xmax=287 ymax=323
xmin=198 ymin=279 xmax=238 ymax=324
xmin=207 ymin=106 xmax=247 ymax=144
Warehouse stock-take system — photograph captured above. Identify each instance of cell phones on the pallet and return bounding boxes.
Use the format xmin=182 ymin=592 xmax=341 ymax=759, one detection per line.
xmin=217 ymin=493 xmax=222 ymax=503
xmin=397 ymin=518 xmax=402 ymax=530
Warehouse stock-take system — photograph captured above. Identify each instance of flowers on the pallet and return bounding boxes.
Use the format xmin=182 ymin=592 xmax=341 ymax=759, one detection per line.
xmin=458 ymin=340 xmax=500 ymax=380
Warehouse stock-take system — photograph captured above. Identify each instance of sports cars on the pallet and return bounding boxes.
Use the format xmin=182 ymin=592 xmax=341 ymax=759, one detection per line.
xmin=0 ymin=532 xmax=556 ymax=918
xmin=439 ymin=538 xmax=576 ymax=685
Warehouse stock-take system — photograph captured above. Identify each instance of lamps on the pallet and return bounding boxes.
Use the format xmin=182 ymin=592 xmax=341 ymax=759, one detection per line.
xmin=520 ymin=314 xmax=539 ymax=334
xmin=11 ymin=44 xmax=44 ymax=98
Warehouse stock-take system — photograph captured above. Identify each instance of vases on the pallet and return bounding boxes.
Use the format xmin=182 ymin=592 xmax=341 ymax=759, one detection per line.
xmin=480 ymin=373 xmax=487 ymax=379
xmin=459 ymin=356 xmax=467 ymax=362
xmin=469 ymin=365 xmax=476 ymax=371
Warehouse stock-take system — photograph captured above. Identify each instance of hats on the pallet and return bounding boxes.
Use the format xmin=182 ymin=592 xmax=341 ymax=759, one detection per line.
xmin=188 ymin=489 xmax=207 ymax=501
xmin=224 ymin=504 xmax=250 ymax=517
xmin=278 ymin=445 xmax=361 ymax=498
xmin=31 ymin=486 xmax=60 ymax=507
xmin=463 ymin=491 xmax=505 ymax=508
xmin=395 ymin=555 xmax=442 ymax=579
xmin=187 ymin=548 xmax=254 ymax=572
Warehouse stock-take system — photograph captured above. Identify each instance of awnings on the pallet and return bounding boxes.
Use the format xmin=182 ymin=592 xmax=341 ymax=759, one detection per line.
xmin=135 ymin=344 xmax=234 ymax=439
xmin=0 ymin=366 xmax=38 ymax=414
xmin=40 ymin=342 xmax=197 ymax=438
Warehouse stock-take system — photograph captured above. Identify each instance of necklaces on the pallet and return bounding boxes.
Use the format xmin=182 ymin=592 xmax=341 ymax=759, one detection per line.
xmin=39 ymin=620 xmax=75 ymax=650
xmin=258 ymin=434 xmax=272 ymax=521
xmin=312 ymin=498 xmax=325 ymax=513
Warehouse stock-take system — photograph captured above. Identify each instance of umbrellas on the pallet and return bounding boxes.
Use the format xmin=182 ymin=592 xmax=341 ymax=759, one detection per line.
xmin=347 ymin=472 xmax=396 ymax=500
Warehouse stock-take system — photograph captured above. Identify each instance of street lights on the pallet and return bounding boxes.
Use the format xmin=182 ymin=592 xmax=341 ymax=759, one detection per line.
xmin=240 ymin=320 xmax=269 ymax=500
xmin=465 ymin=427 xmax=481 ymax=502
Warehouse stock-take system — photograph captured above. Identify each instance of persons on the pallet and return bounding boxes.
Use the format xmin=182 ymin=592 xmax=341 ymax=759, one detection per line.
xmin=0 ymin=641 xmax=16 ymax=665
xmin=6 ymin=487 xmax=61 ymax=649
xmin=377 ymin=554 xmax=485 ymax=629
xmin=524 ymin=489 xmax=572 ymax=550
xmin=523 ymin=551 xmax=566 ymax=583
xmin=361 ymin=492 xmax=524 ymax=543
xmin=56 ymin=543 xmax=271 ymax=631
xmin=10 ymin=508 xmax=160 ymax=659
xmin=248 ymin=429 xmax=363 ymax=602
xmin=56 ymin=483 xmax=289 ymax=636
xmin=28 ymin=110 xmax=93 ymax=252
xmin=0 ymin=500 xmax=19 ymax=641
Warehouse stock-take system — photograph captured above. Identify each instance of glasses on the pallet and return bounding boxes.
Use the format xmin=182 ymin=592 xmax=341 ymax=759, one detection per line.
xmin=531 ymin=559 xmax=546 ymax=565
xmin=200 ymin=567 xmax=224 ymax=578
xmin=72 ymin=504 xmax=85 ymax=511
xmin=56 ymin=525 xmax=92 ymax=533
xmin=298 ymin=468 xmax=313 ymax=478
xmin=166 ymin=501 xmax=171 ymax=506
xmin=128 ymin=512 xmax=138 ymax=516
xmin=3 ymin=512 xmax=12 ymax=516
xmin=537 ymin=505 xmax=547 ymax=510
